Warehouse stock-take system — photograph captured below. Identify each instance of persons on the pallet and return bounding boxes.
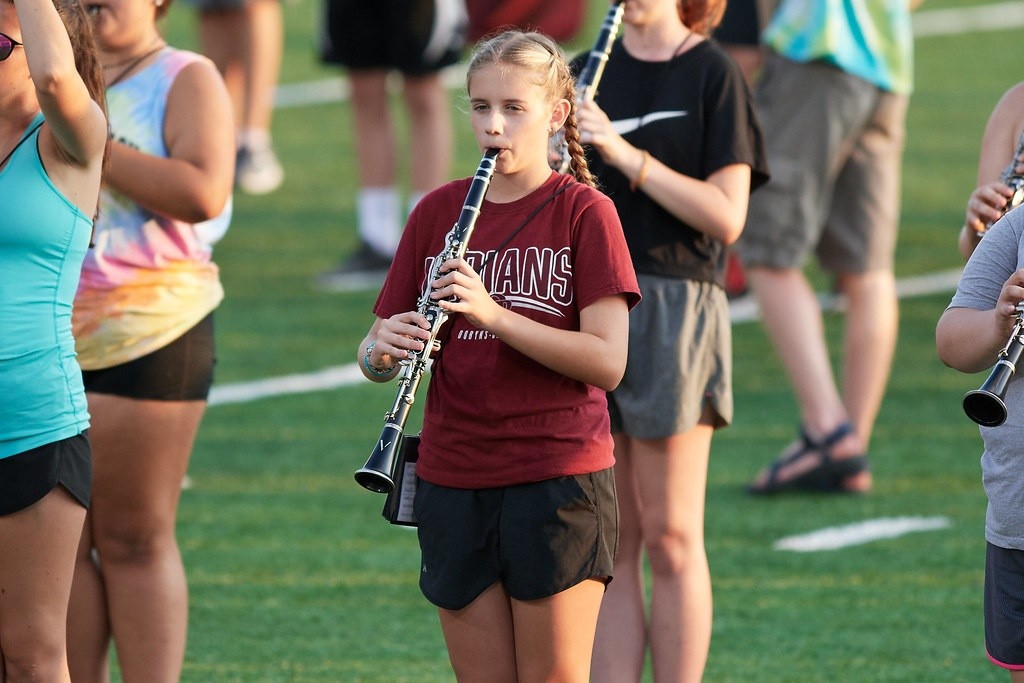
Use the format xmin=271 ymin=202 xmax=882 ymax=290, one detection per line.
xmin=546 ymin=1 xmax=772 ymax=683
xmin=191 ymin=0 xmax=283 ymax=195
xmin=731 ymin=1 xmax=916 ymax=496
xmin=958 ymin=82 xmax=1023 ymax=263
xmin=315 ymin=0 xmax=469 ymax=291
xmin=1 ymin=0 xmax=108 ymax=683
xmin=935 ymin=203 xmax=1024 ymax=683
xmin=356 ymin=30 xmax=644 ymax=683
xmin=65 ymin=0 xmax=237 ymax=683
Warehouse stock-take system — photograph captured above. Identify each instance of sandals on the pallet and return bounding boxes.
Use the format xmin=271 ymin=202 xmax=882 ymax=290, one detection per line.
xmin=800 ymin=457 xmax=871 ymax=494
xmin=747 ymin=421 xmax=863 ymax=497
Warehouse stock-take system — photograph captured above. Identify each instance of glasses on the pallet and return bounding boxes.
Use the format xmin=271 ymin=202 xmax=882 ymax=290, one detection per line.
xmin=0 ymin=32 xmax=23 ymax=61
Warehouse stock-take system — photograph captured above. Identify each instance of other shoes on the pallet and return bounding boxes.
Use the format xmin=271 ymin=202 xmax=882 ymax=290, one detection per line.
xmin=311 ymin=240 xmax=393 ymax=294
xmin=238 ymin=142 xmax=281 ymax=194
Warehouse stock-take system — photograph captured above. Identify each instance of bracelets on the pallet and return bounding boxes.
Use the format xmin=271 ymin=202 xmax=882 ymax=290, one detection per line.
xmin=363 ymin=342 xmax=394 ymax=377
xmin=631 ymin=149 xmax=648 ymax=189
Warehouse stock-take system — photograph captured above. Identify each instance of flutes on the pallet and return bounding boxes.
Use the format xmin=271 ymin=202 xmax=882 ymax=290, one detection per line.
xmin=350 ymin=147 xmax=501 ymax=497
xmin=552 ymin=0 xmax=628 ymax=177
xmin=974 ymin=128 xmax=1024 ymax=238
xmin=960 ymin=296 xmax=1024 ymax=428
